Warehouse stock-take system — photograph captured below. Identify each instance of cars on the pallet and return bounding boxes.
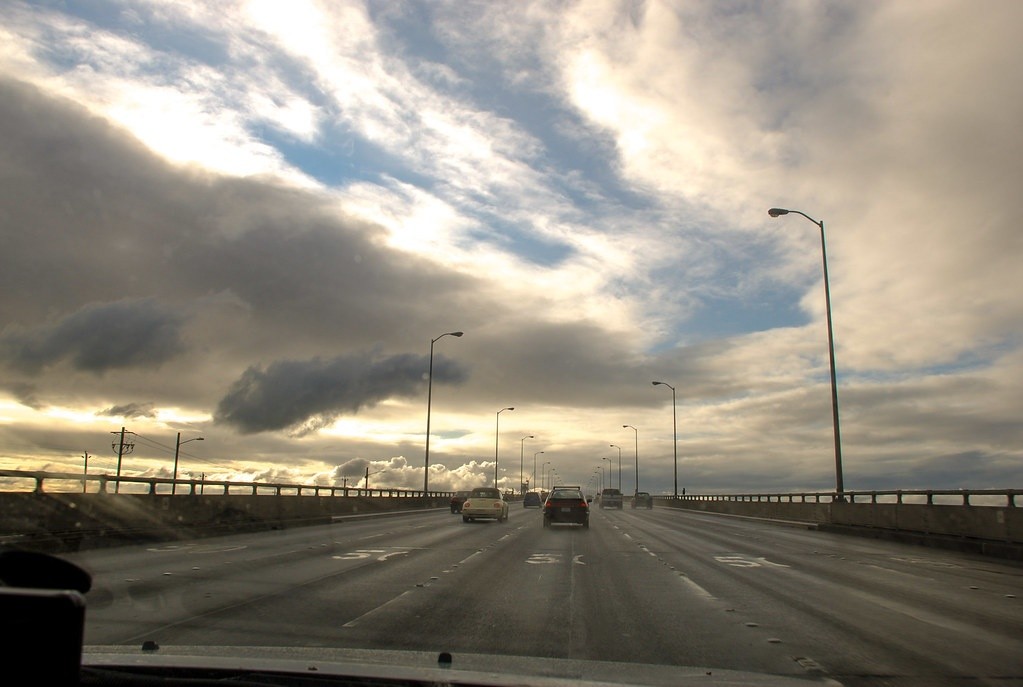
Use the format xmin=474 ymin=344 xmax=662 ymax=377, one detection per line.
xmin=462 ymin=487 xmax=509 ymax=523
xmin=586 ymin=492 xmax=601 ymax=504
xmin=451 ymin=491 xmax=473 ymax=514
xmin=523 ymin=492 xmax=542 ymax=508
xmin=631 ymin=492 xmax=652 ymax=510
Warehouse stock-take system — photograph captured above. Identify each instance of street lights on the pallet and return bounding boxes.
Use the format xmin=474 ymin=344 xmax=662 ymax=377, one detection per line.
xmin=422 ymin=332 xmax=465 ymax=498
xmin=767 ymin=207 xmax=845 ymax=501
xmin=533 ymin=451 xmax=544 ymax=492
xmin=652 ymin=381 xmax=678 ymax=498
xmin=170 ymin=433 xmax=205 ymax=496
xmin=542 ymin=462 xmax=564 ymax=492
xmin=364 ymin=466 xmax=386 ymax=497
xmin=622 ymin=425 xmax=639 ymax=495
xmin=494 ymin=407 xmax=515 ymax=489
xmin=589 ymin=457 xmax=611 ymax=495
xmin=610 ymin=444 xmax=621 ymax=492
xmin=520 ymin=436 xmax=535 ymax=493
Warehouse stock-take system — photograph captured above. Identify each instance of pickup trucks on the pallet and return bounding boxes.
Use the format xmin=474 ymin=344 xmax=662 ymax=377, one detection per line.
xmin=597 ymin=488 xmax=624 ymax=510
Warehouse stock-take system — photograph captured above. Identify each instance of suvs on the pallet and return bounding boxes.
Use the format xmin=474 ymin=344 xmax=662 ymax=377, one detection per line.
xmin=543 ymin=485 xmax=590 ymax=529
